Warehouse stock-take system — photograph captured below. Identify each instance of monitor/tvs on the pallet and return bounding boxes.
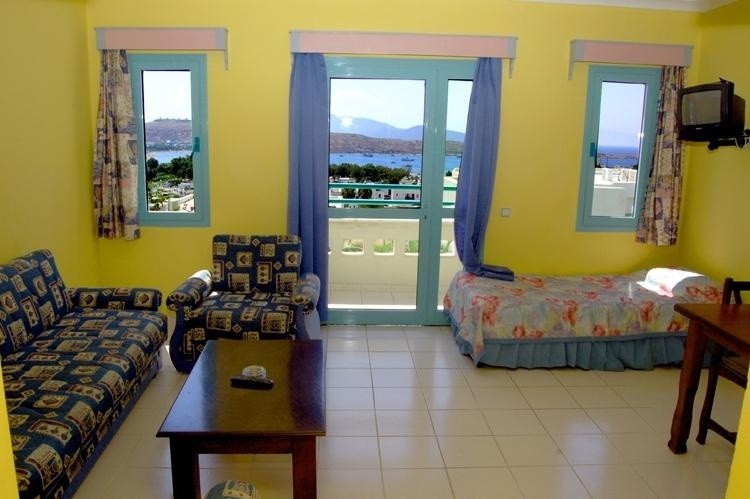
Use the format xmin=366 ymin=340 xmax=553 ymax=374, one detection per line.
xmin=677 ymin=80 xmax=744 ymax=140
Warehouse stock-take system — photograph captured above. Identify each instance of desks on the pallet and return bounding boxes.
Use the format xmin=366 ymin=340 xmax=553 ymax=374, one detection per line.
xmin=668 ymin=304 xmax=750 ymax=454
xmin=156 ymin=338 xmax=325 ymax=498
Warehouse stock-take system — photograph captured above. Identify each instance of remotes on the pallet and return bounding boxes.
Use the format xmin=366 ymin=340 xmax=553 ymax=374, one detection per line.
xmin=229 ymin=374 xmax=274 ymax=390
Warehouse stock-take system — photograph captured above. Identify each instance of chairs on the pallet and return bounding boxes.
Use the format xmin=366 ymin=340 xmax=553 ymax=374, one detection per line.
xmin=166 ymin=234 xmax=322 ymax=373
xmin=696 ymin=278 xmax=750 ymax=445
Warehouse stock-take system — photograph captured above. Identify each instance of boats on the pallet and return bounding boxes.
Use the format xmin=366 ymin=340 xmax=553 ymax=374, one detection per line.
xmin=405 ymin=164 xmax=412 ymax=167
xmin=401 ymin=157 xmax=414 ymax=161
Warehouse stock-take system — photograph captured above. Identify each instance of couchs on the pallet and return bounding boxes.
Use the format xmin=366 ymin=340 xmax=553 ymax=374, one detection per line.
xmin=0 ymin=249 xmax=166 ymax=498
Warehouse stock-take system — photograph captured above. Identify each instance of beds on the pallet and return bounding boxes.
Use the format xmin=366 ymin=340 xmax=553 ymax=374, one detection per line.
xmin=444 ymin=267 xmax=736 ymax=372
